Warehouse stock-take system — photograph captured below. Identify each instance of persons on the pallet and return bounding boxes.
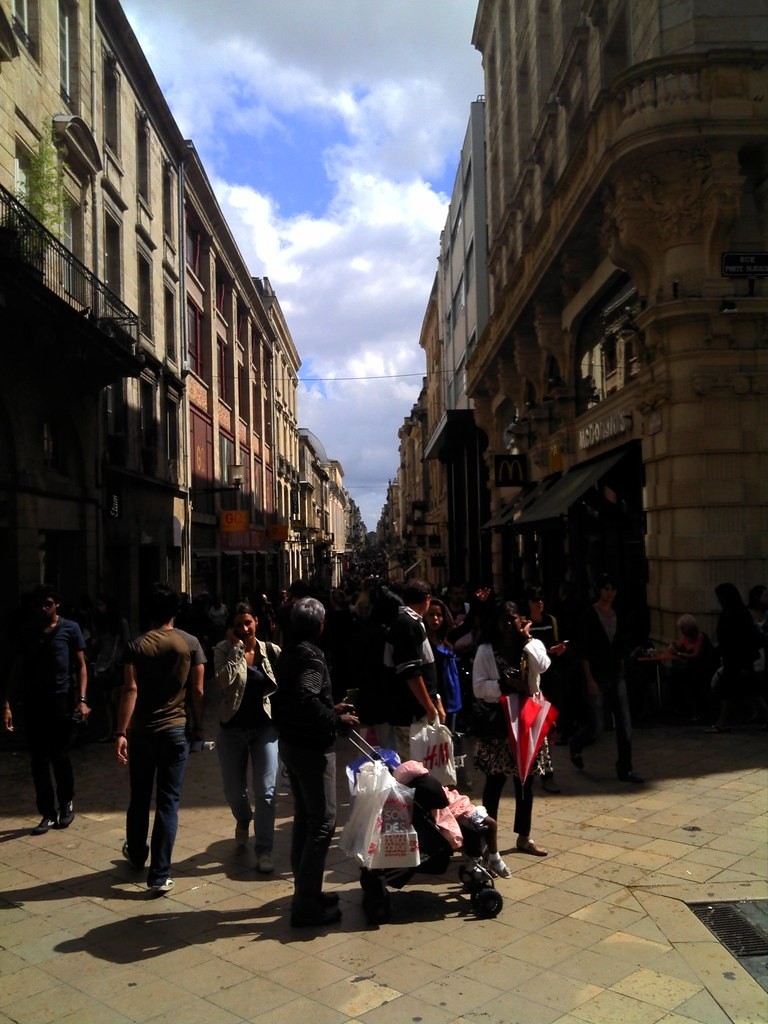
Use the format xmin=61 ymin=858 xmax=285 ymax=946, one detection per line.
xmin=2 ymin=585 xmax=91 ymax=834
xmin=705 ymin=583 xmax=768 ymax=733
xmin=669 ymin=613 xmax=715 ymax=720
xmin=90 ymin=574 xmax=646 ymax=892
xmin=280 ymin=596 xmax=360 ymax=929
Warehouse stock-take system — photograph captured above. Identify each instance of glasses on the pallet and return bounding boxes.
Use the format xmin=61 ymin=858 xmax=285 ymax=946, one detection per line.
xmin=41 ymin=600 xmax=55 ymax=607
xmin=531 ymin=595 xmax=545 ymax=603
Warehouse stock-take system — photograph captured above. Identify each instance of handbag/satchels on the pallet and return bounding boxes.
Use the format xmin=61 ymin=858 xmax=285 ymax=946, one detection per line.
xmin=94 ymin=664 xmax=113 ymax=686
xmin=339 ymin=749 xmax=421 ymax=871
xmin=409 ymin=715 xmax=458 ymax=787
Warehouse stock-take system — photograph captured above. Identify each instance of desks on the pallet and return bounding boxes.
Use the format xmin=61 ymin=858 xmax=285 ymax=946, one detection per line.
xmin=638 ymin=649 xmax=680 ymax=716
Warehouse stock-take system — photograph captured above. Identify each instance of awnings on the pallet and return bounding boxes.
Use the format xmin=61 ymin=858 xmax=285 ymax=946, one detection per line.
xmin=507 ymin=451 xmax=626 ymax=526
xmin=479 ymin=474 xmax=554 ymax=530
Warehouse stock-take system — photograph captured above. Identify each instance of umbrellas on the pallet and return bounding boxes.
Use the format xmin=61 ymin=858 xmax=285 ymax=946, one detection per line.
xmin=501 ymin=693 xmax=560 ymax=799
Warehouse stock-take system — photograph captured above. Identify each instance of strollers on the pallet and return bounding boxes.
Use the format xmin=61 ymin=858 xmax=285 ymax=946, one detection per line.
xmin=340 ymin=706 xmax=504 ymax=925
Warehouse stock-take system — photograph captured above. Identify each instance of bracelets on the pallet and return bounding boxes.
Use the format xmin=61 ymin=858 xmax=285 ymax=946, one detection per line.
xmin=677 ymin=652 xmax=681 ymax=655
xmin=116 ymin=732 xmax=126 ymax=737
xmin=77 ymin=697 xmax=88 ymax=703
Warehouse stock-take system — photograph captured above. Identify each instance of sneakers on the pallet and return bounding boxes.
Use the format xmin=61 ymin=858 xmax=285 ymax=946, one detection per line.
xmin=59 ymin=800 xmax=75 ymax=827
xmin=33 ymin=812 xmax=59 ymax=835
xmin=122 ymin=840 xmax=145 ymax=870
xmin=151 ymin=878 xmax=176 ymax=892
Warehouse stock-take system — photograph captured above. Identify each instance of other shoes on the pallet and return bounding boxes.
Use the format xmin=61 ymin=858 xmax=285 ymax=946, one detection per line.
xmin=570 ymin=743 xmax=584 ymax=769
xmin=235 ymin=823 xmax=249 ymax=846
xmin=290 ymin=892 xmax=343 ymax=926
xmin=704 ymin=725 xmax=730 ymax=734
xmin=516 ymin=834 xmax=548 ymax=857
xmin=256 ymin=854 xmax=274 ymax=872
xmin=543 ymin=779 xmax=560 ymax=793
xmin=617 ymin=770 xmax=644 ymax=784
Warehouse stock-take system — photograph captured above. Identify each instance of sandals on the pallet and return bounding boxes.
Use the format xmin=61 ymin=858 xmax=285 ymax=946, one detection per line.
xmin=482 ymin=857 xmax=511 ymax=879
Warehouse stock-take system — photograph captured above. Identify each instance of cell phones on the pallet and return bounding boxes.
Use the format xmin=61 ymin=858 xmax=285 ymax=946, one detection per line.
xmin=233 ymin=630 xmax=241 ymax=638
